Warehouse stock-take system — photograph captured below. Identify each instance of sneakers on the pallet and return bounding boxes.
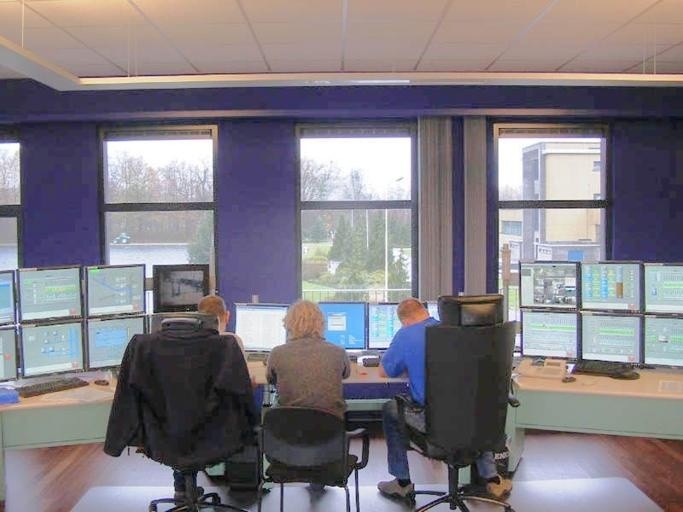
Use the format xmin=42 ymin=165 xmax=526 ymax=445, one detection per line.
xmin=175 ymin=487 xmax=204 ymax=498
xmin=486 ymin=474 xmax=513 ymax=498
xmin=378 ymin=478 xmax=416 ymax=498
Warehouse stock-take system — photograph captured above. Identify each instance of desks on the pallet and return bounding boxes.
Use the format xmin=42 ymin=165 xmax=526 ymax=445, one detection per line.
xmin=0 ymin=358 xmax=682 ymax=452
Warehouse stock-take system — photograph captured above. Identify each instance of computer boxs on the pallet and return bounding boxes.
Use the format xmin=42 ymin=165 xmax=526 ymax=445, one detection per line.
xmin=224 ymin=444 xmax=260 ymax=491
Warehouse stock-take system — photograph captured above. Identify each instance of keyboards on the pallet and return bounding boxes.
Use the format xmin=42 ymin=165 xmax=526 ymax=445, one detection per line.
xmin=573 ymin=361 xmax=625 ymax=375
xmin=363 ymin=358 xmax=381 ymax=367
xmin=349 ymin=355 xmax=357 ymax=364
xmin=14 ymin=377 xmax=89 ymax=398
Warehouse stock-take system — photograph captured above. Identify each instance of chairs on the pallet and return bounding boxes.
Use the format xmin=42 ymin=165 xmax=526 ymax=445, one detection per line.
xmin=257 ymin=405 xmax=369 ymax=512
xmin=149 ymin=310 xmax=252 ymax=512
xmin=403 ymin=293 xmax=519 ymax=512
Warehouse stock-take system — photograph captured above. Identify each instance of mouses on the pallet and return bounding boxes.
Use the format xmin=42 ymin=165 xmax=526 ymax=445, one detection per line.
xmin=95 ymin=380 xmax=109 ymax=386
xmin=562 ymin=376 xmax=576 ymax=383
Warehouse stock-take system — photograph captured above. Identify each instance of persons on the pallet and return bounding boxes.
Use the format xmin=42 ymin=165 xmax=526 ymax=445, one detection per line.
xmin=199 ymin=296 xmax=245 ymax=359
xmin=377 ymin=298 xmax=513 ymax=499
xmin=265 ymin=301 xmax=351 ymax=423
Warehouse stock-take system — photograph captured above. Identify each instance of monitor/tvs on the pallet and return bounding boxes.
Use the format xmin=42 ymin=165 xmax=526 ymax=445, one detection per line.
xmin=233 ymin=303 xmax=290 ymax=362
xmin=518 ymin=260 xmax=682 ymax=369
xmin=367 ymin=302 xmax=427 ymax=359
xmin=152 ymin=263 xmax=211 ymax=314
xmin=316 ymin=302 xmax=366 ymax=351
xmin=0 ymin=264 xmax=151 ymax=382
xmin=425 ymin=300 xmax=440 ymax=322
